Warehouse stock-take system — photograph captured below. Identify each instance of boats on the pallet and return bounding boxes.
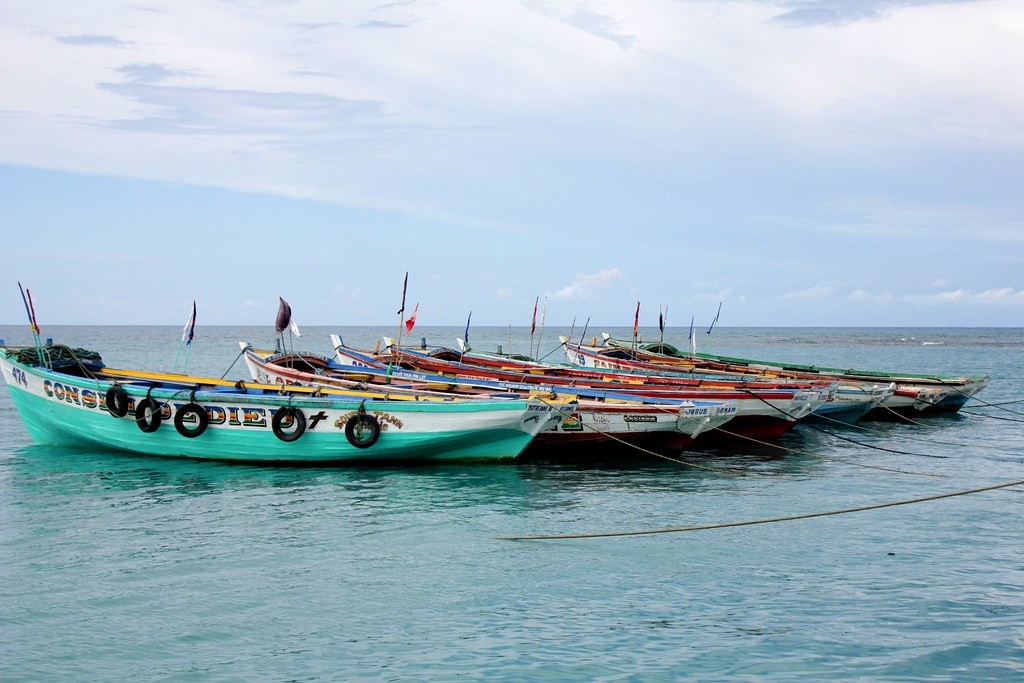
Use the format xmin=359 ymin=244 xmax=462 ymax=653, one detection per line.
xmin=329 ymin=330 xmax=994 ymax=451
xmin=1 ymin=337 xmax=563 ymax=467
xmin=237 ymin=338 xmax=740 ymax=468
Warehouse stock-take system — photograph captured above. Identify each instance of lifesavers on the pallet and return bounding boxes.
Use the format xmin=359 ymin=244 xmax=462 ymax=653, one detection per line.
xmin=133 ymin=396 xmax=163 ymax=434
xmin=343 ymin=413 xmax=382 ymax=450
xmin=104 ymin=385 xmax=130 ymax=419
xmin=269 ymin=403 xmax=308 ymax=445
xmin=172 ymin=402 xmax=211 ymax=439
